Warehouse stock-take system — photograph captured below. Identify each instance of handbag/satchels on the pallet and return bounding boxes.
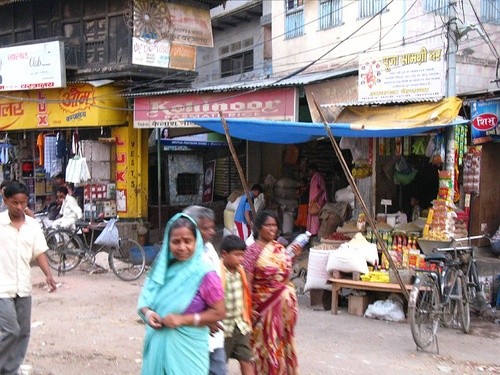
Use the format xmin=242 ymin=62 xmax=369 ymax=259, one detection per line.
xmin=308 ymin=201 xmax=321 ymax=215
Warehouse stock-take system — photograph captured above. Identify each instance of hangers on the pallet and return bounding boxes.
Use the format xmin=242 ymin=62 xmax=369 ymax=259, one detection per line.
xmin=44 ymin=129 xmax=57 ymax=137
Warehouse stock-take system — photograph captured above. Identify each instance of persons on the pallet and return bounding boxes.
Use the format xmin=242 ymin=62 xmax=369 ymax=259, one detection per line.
xmin=206 ymin=170 xmax=212 ymax=184
xmin=234 ymin=183 xmax=262 ymax=242
xmin=240 ymin=211 xmax=301 ymax=375
xmin=0 ymin=180 xmax=57 ymax=375
xmin=294 ymin=179 xmax=309 ymax=233
xmin=306 ymin=163 xmax=328 ymax=236
xmin=219 ymin=235 xmax=255 ymax=375
xmin=490 ymin=226 xmax=500 ymax=258
xmin=410 ymin=160 xmax=439 ymax=218
xmin=51 ymin=172 xmax=75 ymax=220
xmin=137 ymin=213 xmax=226 ymax=375
xmin=331 ymin=157 xmax=349 ymax=200
xmin=376 ymin=157 xmax=400 ymax=213
xmin=183 ymin=205 xmax=227 ymax=375
xmin=161 ymin=128 xmax=168 ymax=139
xmin=51 ymin=186 xmax=82 ymax=249
xmin=252 ymin=187 xmax=272 ymax=213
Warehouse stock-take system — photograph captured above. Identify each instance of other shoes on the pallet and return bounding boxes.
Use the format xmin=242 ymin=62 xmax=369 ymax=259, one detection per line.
xmin=364 ymin=293 xmax=406 ymax=323
xmin=56 ymin=242 xmax=63 ymax=247
xmin=70 ymin=244 xmax=76 ymax=250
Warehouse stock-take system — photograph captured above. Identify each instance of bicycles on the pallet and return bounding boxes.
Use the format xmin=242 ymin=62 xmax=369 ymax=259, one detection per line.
xmin=33 ymin=211 xmax=67 ymax=264
xmin=406 ymin=231 xmax=493 ymax=356
xmin=43 ymin=212 xmax=147 ymax=282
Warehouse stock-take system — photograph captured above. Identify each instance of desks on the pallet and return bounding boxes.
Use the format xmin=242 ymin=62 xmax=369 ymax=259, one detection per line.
xmin=327 ymin=279 xmax=450 ymax=319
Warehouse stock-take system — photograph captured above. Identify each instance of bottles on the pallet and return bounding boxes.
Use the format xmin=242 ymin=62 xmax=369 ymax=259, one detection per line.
xmin=285 ymin=230 xmax=312 ymax=257
xmin=372 ymin=260 xmax=379 ymax=272
xmin=356 ymin=213 xmax=417 ymax=251
xmin=380 ymin=258 xmax=386 ymax=272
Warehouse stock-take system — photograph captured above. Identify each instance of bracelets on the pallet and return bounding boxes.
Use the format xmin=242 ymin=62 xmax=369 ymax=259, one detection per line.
xmin=145 ymin=310 xmax=154 ymax=318
xmin=194 ymin=313 xmax=200 ymax=327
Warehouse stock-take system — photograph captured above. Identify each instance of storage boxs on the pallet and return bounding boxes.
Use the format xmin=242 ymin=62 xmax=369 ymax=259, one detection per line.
xmin=129 ymin=246 xmax=160 ymax=265
xmin=348 ymin=295 xmax=370 ymax=315
